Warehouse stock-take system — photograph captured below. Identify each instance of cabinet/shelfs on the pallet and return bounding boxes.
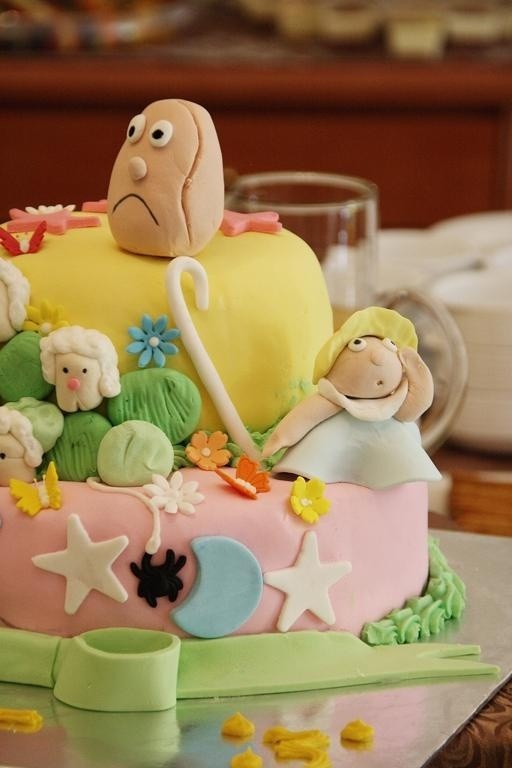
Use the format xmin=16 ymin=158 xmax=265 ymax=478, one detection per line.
xmin=1 ymin=15 xmax=510 ymax=236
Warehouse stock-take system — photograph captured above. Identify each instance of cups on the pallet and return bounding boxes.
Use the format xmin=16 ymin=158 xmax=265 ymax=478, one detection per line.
xmin=230 ymin=168 xmax=468 ymax=460
xmin=426 ymin=265 xmax=511 ymax=459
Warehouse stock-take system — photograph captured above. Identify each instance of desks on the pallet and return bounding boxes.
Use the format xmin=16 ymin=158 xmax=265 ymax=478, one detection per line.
xmin=398 ymin=443 xmax=510 ymax=768
